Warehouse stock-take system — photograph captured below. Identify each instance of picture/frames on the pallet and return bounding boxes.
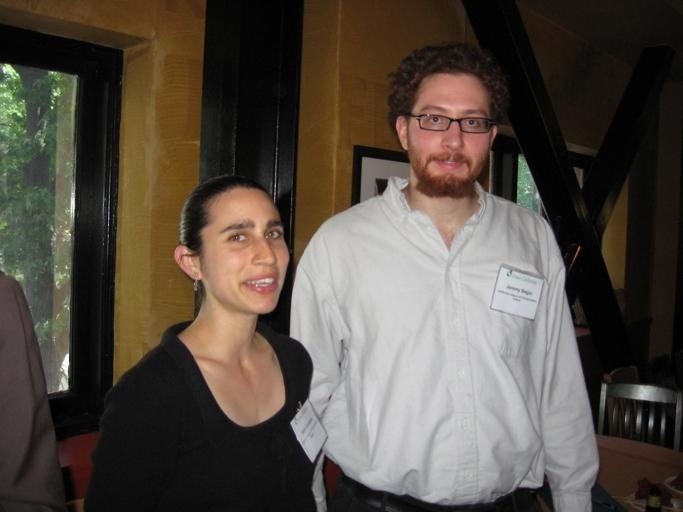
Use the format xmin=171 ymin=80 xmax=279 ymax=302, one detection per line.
xmin=350 ymin=143 xmax=411 ymax=207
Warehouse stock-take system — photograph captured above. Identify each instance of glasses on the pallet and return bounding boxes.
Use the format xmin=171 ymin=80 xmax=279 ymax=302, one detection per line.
xmin=404 ymin=111 xmax=498 ymax=133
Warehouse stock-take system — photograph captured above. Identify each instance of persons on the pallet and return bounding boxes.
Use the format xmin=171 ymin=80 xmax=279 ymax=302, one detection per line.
xmin=84 ymin=176 xmax=323 ymax=512
xmin=289 ymin=43 xmax=599 ymax=512
xmin=0 ymin=271 xmax=67 ymax=512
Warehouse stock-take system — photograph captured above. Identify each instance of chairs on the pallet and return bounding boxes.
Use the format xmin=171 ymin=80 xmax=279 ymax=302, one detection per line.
xmin=599 ymin=364 xmax=683 ymax=451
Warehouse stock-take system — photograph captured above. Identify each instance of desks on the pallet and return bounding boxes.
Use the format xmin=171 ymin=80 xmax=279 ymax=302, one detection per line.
xmin=595 ymin=432 xmax=683 ymax=512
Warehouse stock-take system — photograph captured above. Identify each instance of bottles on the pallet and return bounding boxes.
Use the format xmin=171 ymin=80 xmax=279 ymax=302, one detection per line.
xmin=644 ymin=484 xmax=666 ymax=511
